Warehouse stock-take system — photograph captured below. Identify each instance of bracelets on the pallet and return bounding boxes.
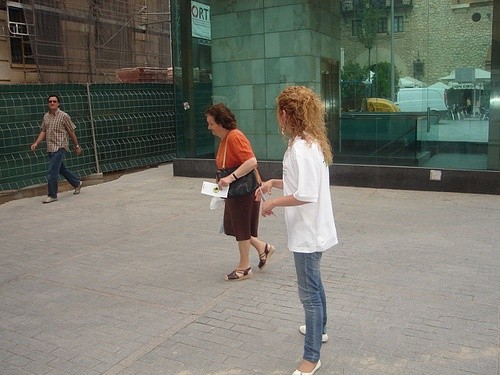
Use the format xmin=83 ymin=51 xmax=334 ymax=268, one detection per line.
xmin=232 ymin=173 xmax=238 ymax=180
xmin=74 ymin=144 xmax=80 ymax=148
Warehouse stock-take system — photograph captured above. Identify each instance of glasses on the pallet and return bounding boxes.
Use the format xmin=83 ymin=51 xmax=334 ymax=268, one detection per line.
xmin=48 ymin=100 xmax=58 ymax=103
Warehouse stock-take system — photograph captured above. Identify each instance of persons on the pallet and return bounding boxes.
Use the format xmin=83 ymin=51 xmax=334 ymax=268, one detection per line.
xmin=206 ymin=103 xmax=275 ymax=281
xmin=467 ymin=96 xmax=472 ymax=115
xmin=31 ymin=94 xmax=83 ymax=204
xmin=255 ymin=85 xmax=338 ymax=375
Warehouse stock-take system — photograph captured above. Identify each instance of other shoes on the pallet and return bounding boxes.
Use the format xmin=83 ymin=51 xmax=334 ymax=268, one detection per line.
xmin=293 ymin=359 xmax=321 ymax=375
xmin=43 ymin=197 xmax=58 ymax=203
xmin=300 ymin=325 xmax=328 ymax=342
xmin=74 ymin=181 xmax=83 ymax=194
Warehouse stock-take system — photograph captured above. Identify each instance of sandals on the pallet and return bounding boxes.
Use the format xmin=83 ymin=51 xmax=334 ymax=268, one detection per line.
xmin=223 ymin=267 xmax=253 ymax=281
xmin=258 ymin=244 xmax=275 ymax=270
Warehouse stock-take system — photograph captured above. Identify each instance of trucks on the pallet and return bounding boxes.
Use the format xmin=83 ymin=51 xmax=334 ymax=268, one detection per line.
xmin=396 ymin=85 xmax=451 ymax=125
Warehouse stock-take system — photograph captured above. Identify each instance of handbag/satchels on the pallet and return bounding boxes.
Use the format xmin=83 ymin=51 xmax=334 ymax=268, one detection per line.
xmin=216 ymin=132 xmax=260 ymax=199
